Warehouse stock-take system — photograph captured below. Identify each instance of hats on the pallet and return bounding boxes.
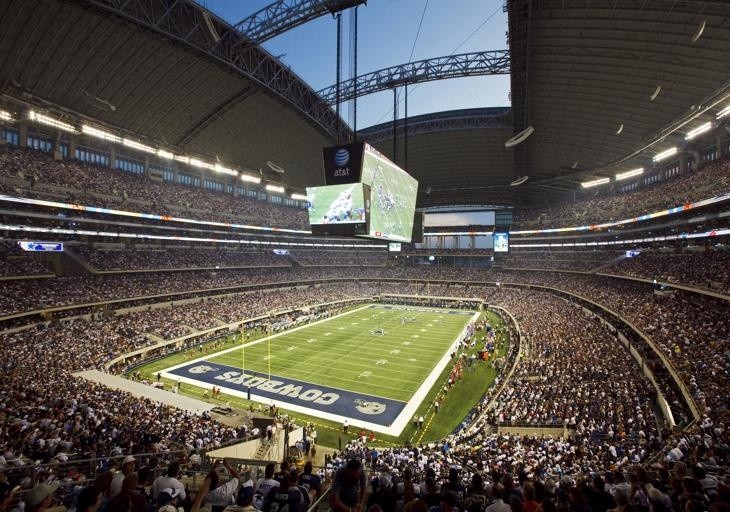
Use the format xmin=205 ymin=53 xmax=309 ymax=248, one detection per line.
xmin=25 ymin=480 xmax=60 ymax=505
xmin=264 ymin=462 xmax=275 ymax=477
xmin=157 ymin=486 xmax=182 ymax=506
xmin=121 ymin=455 xmax=137 ymax=468
xmin=237 ymin=479 xmax=254 ymax=507
